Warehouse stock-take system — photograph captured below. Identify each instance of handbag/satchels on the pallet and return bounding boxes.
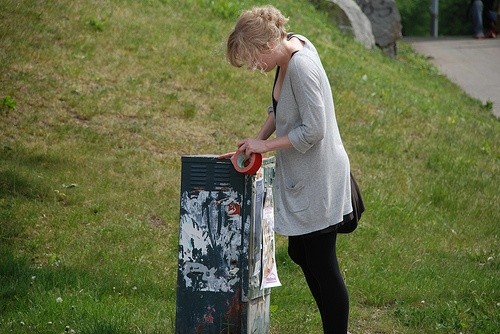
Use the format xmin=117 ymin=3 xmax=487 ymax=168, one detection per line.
xmin=337 ymin=172 xmax=365 ymax=233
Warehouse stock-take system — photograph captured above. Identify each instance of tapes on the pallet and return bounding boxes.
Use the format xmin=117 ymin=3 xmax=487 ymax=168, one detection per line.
xmin=230 ymin=149 xmax=263 ymax=177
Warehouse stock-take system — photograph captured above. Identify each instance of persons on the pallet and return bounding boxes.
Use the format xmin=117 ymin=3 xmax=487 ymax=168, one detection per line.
xmin=219 ymin=3 xmax=356 ymax=334
